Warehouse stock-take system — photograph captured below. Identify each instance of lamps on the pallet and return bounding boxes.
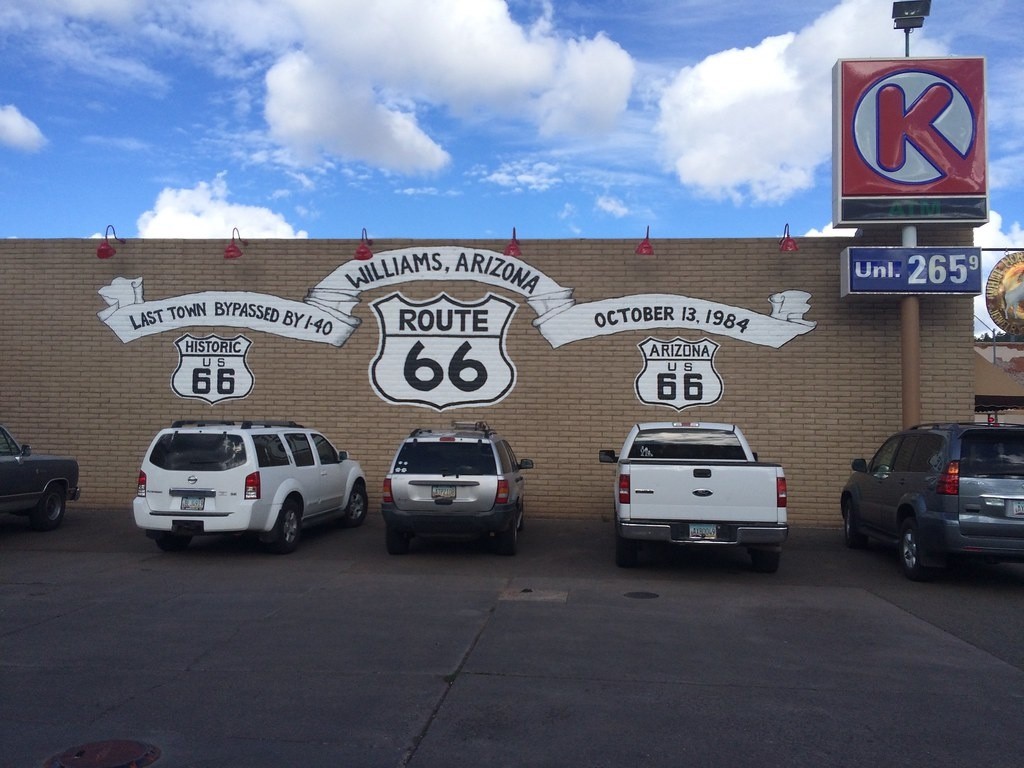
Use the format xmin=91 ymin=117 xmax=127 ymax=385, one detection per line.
xmin=634 ymin=225 xmax=655 ymax=256
xmin=503 ymin=226 xmax=523 ymax=256
xmin=97 ymin=226 xmax=125 ymax=259
xmin=778 ymin=224 xmax=798 ymax=251
xmin=354 ymin=228 xmax=372 ymax=260
xmin=224 ymin=227 xmax=248 ymax=259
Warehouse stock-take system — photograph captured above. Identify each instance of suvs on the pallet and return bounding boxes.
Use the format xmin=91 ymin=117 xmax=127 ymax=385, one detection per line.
xmin=379 ymin=421 xmax=534 ymax=552
xmin=132 ymin=416 xmax=370 ymax=554
xmin=838 ymin=419 xmax=1024 ymax=580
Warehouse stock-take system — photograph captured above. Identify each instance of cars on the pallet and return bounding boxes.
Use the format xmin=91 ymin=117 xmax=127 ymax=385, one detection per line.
xmin=0 ymin=426 xmax=81 ymax=529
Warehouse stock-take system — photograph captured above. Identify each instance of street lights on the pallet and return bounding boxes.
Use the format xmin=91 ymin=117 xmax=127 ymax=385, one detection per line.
xmin=894 ymin=0 xmax=932 ymax=432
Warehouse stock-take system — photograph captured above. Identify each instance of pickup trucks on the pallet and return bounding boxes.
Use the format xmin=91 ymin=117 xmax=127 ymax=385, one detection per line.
xmin=599 ymin=421 xmax=791 ymax=573
